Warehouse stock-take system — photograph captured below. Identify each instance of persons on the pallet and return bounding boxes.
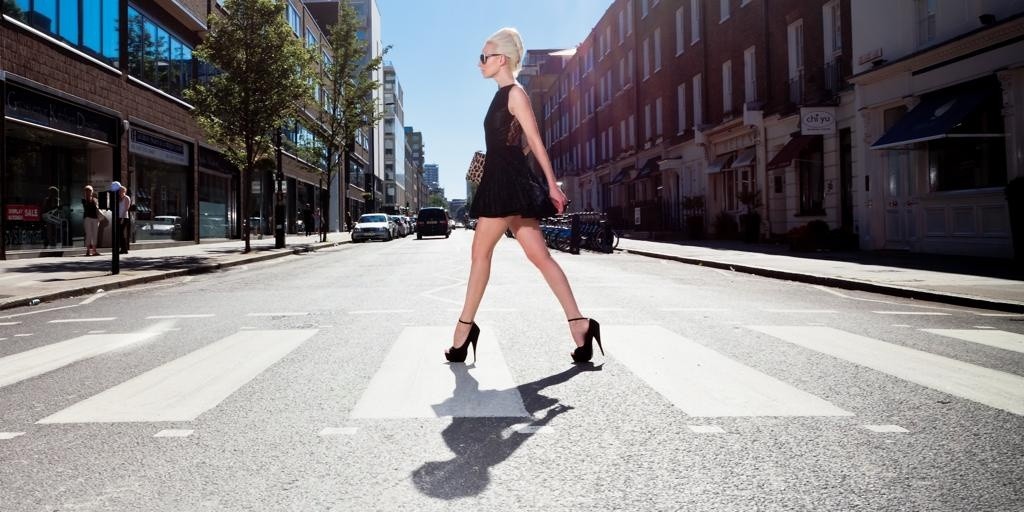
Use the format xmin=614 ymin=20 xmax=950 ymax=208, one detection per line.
xmin=346 ymin=211 xmax=353 ymax=233
xmin=118 ymin=186 xmax=131 ymax=254
xmin=303 ymin=203 xmax=313 ymax=236
xmin=312 ymin=208 xmax=325 ymax=234
xmin=81 ymin=185 xmax=100 ymax=256
xmin=40 ymin=187 xmax=59 ymax=248
xmin=445 ymin=27 xmax=604 ymax=362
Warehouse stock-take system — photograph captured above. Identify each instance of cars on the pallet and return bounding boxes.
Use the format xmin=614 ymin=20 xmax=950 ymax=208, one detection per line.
xmin=137 ymin=214 xmax=306 ymax=239
xmin=349 ymin=205 xmax=476 ymax=243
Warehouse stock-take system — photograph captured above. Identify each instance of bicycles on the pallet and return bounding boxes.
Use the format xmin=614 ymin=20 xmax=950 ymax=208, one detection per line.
xmin=504 ymin=198 xmax=620 ymax=252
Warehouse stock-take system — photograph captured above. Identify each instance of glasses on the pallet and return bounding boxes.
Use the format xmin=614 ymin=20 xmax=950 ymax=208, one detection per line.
xmin=480 ymin=53 xmax=510 ymax=64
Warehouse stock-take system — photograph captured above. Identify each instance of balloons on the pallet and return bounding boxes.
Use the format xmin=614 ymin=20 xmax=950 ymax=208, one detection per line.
xmin=111 ymin=180 xmax=121 ymax=192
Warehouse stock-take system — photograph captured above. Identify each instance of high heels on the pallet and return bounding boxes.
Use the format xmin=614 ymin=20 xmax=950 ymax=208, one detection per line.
xmin=568 ymin=318 xmax=604 ymax=362
xmin=445 ymin=319 xmax=480 ymax=362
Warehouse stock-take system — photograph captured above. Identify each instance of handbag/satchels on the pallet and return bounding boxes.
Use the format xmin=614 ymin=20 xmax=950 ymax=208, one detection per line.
xmin=466 ymin=150 xmax=487 ymax=185
